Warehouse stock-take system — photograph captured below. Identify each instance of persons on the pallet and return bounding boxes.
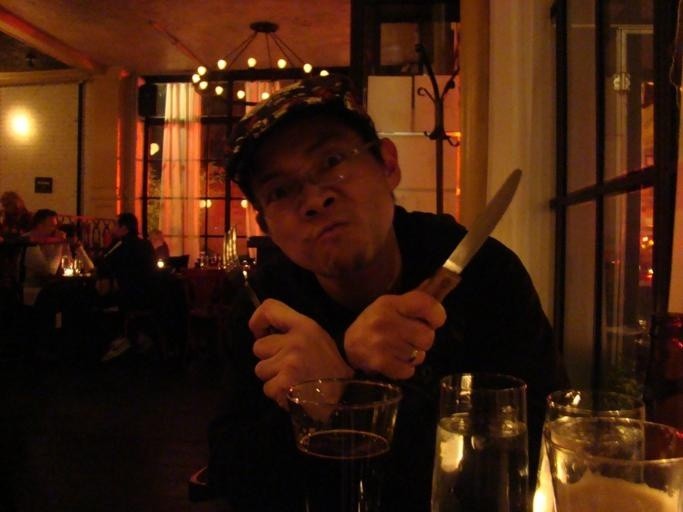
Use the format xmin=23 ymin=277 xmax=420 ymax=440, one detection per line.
xmin=207 ymin=83 xmax=572 ymax=512
xmin=21 ymin=208 xmax=160 ymax=362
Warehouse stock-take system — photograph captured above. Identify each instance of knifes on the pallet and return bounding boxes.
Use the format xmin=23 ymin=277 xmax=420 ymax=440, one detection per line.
xmin=421 ymin=168 xmax=522 ymax=304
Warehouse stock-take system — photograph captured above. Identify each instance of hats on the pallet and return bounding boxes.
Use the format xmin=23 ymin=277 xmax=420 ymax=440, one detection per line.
xmin=229 ymin=78 xmax=373 ymax=183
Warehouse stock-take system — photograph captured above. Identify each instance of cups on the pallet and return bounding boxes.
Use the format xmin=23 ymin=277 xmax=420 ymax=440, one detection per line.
xmin=542 ymin=417 xmax=681 ymax=512
xmin=288 ymin=376 xmax=404 ymax=509
xmin=531 ymin=389 xmax=645 ymax=511
xmin=428 ymin=374 xmax=531 ymax=511
xmin=59 ymin=255 xmax=83 ymax=276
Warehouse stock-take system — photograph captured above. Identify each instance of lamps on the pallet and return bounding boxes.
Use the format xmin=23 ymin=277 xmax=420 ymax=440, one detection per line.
xmin=191 ymin=20 xmax=330 ymax=107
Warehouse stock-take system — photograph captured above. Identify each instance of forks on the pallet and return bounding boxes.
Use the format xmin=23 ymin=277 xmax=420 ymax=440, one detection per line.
xmin=222 ymin=224 xmax=260 ymax=309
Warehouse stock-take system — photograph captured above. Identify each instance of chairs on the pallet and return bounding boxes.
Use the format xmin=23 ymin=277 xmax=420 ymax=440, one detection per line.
xmin=1 ymin=214 xmax=254 ymax=364
